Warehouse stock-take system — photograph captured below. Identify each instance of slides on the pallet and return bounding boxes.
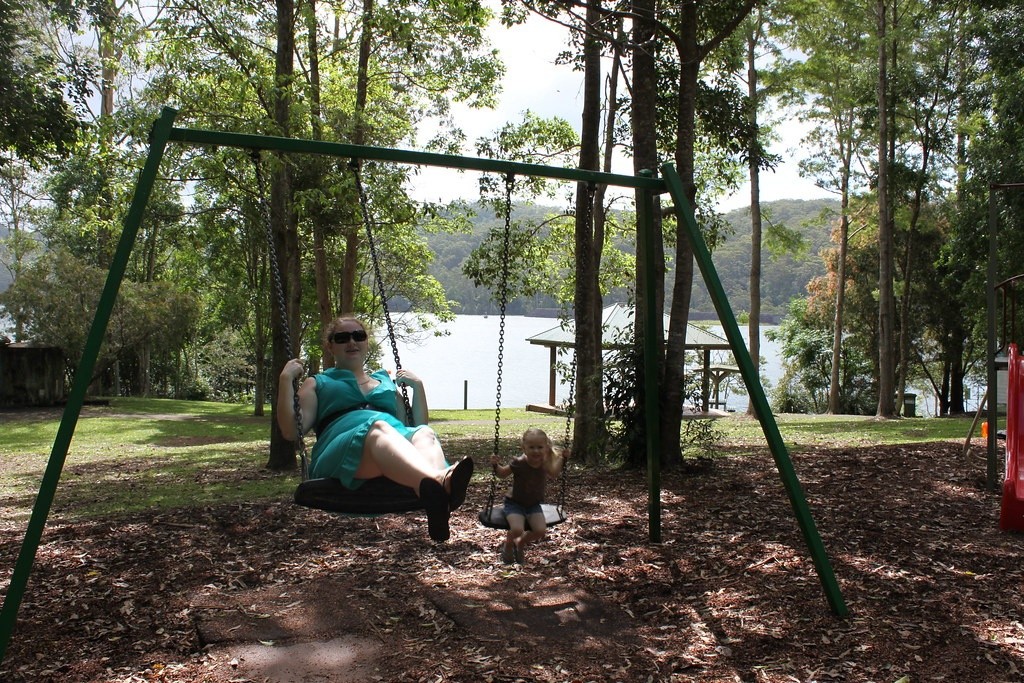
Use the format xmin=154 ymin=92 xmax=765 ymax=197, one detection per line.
xmin=1001 ymin=343 xmax=1024 ymax=530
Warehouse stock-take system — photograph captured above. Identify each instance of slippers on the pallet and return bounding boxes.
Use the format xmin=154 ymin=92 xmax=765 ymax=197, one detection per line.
xmin=503 ymin=545 xmax=525 ymax=565
xmin=419 ymin=456 xmax=475 ymax=542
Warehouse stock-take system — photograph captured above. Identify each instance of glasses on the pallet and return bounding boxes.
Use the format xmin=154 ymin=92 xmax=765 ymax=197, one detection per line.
xmin=332 ymin=329 xmax=368 ymax=344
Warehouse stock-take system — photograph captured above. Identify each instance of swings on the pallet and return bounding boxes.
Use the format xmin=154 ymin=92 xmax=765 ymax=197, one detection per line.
xmin=479 ymin=190 xmax=590 ymax=531
xmin=254 ymin=160 xmax=426 ymax=514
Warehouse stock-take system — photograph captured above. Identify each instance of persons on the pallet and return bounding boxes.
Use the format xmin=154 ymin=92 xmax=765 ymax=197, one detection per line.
xmin=276 ymin=315 xmax=473 ymax=542
xmin=489 ymin=428 xmax=570 ymax=563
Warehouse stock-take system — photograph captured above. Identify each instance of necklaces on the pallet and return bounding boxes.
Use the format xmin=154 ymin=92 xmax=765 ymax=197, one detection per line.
xmin=358 ymin=376 xmax=370 ymax=385
